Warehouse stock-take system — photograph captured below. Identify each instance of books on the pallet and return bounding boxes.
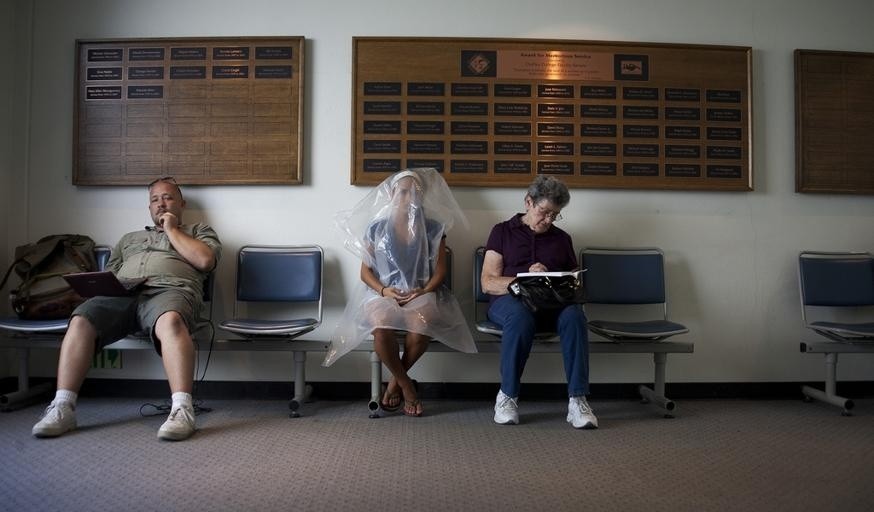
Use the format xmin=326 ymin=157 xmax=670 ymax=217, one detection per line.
xmin=517 ymin=269 xmax=588 ymax=279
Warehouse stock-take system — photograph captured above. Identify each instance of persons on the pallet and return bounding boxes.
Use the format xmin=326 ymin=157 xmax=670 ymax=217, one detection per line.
xmin=480 ymin=175 xmax=599 ymax=430
xmin=32 ymin=176 xmax=221 ymax=441
xmin=361 ymin=169 xmax=447 ymax=419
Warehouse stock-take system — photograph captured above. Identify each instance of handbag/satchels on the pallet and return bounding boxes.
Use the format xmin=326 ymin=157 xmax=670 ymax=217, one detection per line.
xmin=506 ymin=264 xmax=588 ymax=314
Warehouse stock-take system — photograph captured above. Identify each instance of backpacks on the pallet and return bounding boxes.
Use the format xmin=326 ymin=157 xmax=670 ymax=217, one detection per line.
xmin=0 ymin=234 xmax=99 ymax=322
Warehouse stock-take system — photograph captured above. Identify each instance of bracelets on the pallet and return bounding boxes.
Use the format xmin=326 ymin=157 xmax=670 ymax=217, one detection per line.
xmin=381 ymin=287 xmax=385 ymax=298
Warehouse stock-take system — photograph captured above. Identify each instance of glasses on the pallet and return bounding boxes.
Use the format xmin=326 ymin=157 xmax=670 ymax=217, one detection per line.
xmin=147 ymin=177 xmax=183 ymax=200
xmin=529 ymin=195 xmax=562 ymax=221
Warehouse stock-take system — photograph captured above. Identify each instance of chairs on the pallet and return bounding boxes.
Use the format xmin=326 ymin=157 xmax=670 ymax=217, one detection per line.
xmin=0 ymin=245 xmax=115 ymax=331
xmin=797 ymin=248 xmax=873 ymax=346
xmin=218 ymin=244 xmax=324 ymax=345
xmin=124 ymin=271 xmax=216 ymax=340
xmin=360 ymin=246 xmax=456 ymax=336
xmin=473 ymin=245 xmax=564 ymax=346
xmin=579 ymin=245 xmax=689 ymax=342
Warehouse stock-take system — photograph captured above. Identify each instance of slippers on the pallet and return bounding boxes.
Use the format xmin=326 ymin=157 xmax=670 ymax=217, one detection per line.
xmin=379 ymin=381 xmax=403 ymax=412
xmin=401 ymin=379 xmax=425 ymax=417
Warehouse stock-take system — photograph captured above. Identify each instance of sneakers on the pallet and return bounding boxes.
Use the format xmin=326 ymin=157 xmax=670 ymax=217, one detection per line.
xmin=157 ymin=404 xmax=199 ymax=441
xmin=32 ymin=400 xmax=77 ymax=437
xmin=493 ymin=388 xmax=520 ymax=425
xmin=566 ymin=395 xmax=599 ymax=429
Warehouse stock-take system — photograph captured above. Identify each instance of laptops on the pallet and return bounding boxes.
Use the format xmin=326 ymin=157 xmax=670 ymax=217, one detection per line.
xmin=62 ymin=271 xmax=148 ymax=298
